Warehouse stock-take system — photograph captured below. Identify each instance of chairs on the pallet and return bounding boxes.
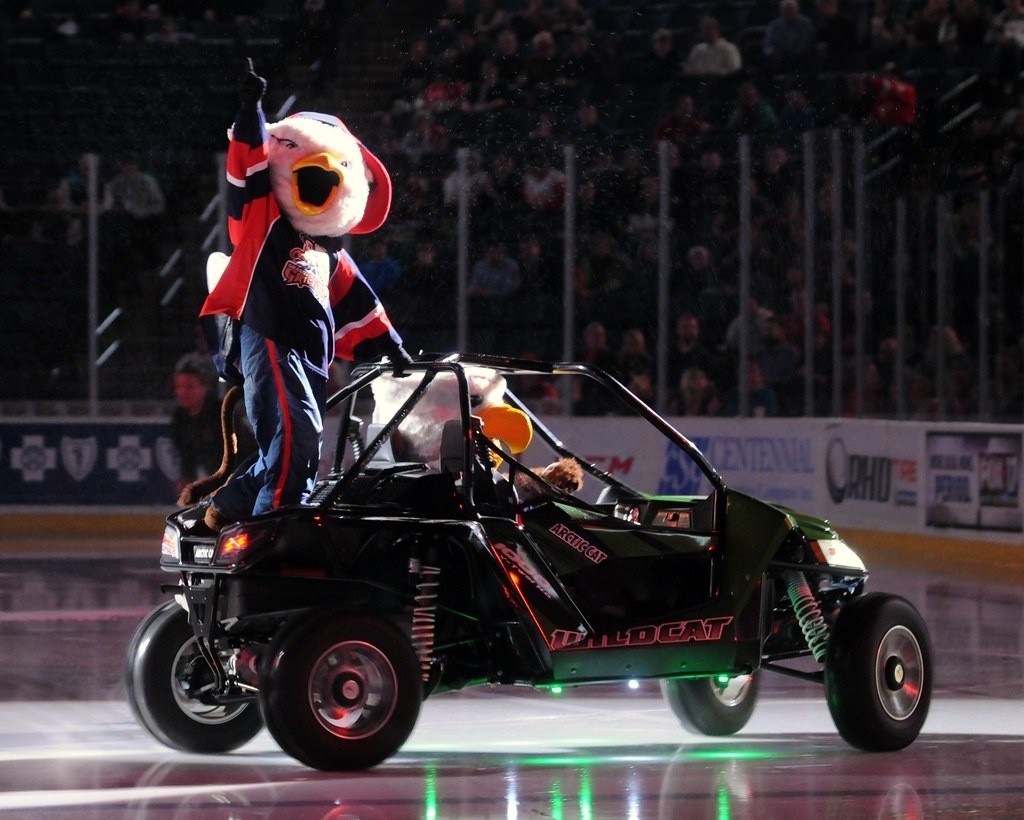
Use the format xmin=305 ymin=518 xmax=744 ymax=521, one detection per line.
xmin=359 ymin=416 xmax=519 ymax=537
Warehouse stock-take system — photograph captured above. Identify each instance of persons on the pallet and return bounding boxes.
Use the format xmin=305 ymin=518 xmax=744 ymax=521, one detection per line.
xmin=170 ymin=350 xmax=225 ymax=496
xmin=0 ymin=0 xmax=1024 ymax=417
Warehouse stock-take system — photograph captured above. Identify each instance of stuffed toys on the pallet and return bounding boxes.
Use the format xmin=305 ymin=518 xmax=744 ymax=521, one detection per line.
xmin=367 ymin=362 xmax=583 ymax=502
xmin=178 ymin=56 xmax=415 ymax=537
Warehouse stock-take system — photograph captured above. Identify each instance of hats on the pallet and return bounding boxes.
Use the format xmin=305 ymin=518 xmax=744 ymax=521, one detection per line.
xmin=654 ymin=28 xmax=672 ymax=41
xmin=119 ymin=149 xmax=138 ymax=164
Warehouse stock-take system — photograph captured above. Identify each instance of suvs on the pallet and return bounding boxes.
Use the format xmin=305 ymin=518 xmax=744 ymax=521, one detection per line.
xmin=122 ymin=353 xmax=937 ymax=774
